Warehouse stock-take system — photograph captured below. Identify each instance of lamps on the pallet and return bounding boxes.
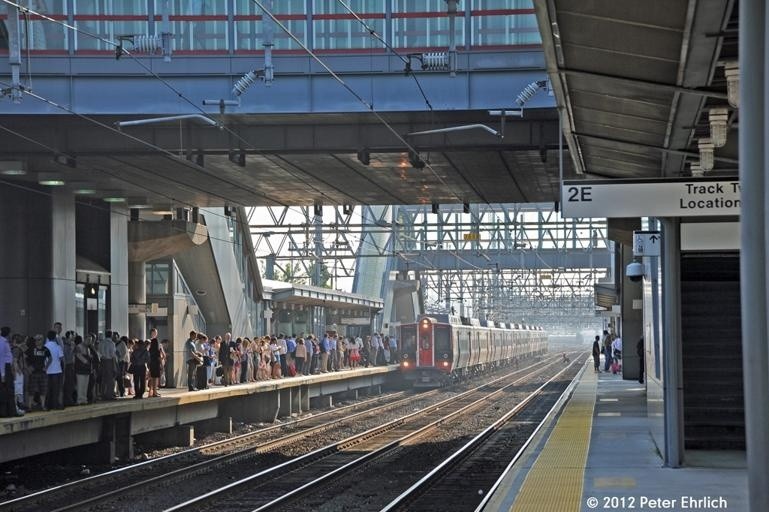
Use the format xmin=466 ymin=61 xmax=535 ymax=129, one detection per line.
xmin=698 ymin=138 xmax=714 ymax=171
xmin=186 ymin=121 xmax=425 ymax=168
xmin=626 ymin=259 xmax=645 ymax=281
xmin=690 ymin=162 xmax=701 ymax=176
xmin=724 ymin=61 xmax=743 ymax=108
xmin=709 ymin=108 xmax=728 ymax=147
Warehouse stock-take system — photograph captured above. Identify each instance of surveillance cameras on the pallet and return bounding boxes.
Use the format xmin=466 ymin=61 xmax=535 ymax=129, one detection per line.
xmin=626 ymin=262 xmax=645 ymax=282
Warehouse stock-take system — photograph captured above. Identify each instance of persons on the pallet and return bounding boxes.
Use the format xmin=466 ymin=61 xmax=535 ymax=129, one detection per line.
xmin=2 ymin=322 xmax=396 ymax=416
xmin=637 ymin=338 xmax=644 ymax=382
xmin=592 ymin=330 xmax=622 ymax=373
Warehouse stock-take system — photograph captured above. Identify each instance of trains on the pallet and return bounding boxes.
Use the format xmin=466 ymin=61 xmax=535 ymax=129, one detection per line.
xmin=397 ymin=310 xmax=549 ymax=390
